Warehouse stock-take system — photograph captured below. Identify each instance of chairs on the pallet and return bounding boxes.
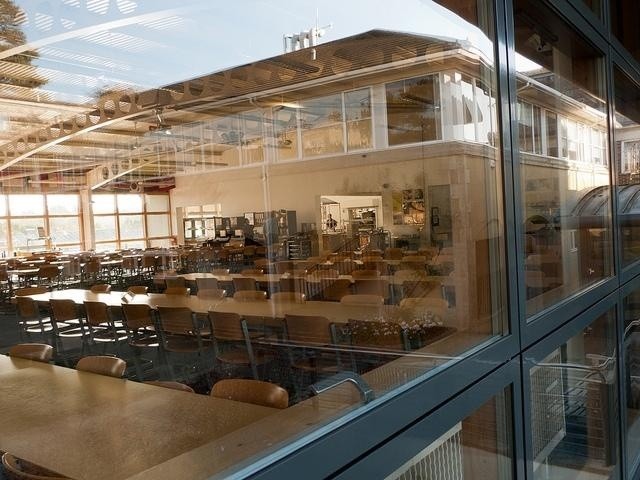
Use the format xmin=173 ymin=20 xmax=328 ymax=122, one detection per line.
xmin=0 ymin=228 xmax=639 ymax=479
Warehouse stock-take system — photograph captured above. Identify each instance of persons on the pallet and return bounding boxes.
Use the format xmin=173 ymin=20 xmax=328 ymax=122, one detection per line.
xmin=326 ymin=213 xmax=337 ymax=232
